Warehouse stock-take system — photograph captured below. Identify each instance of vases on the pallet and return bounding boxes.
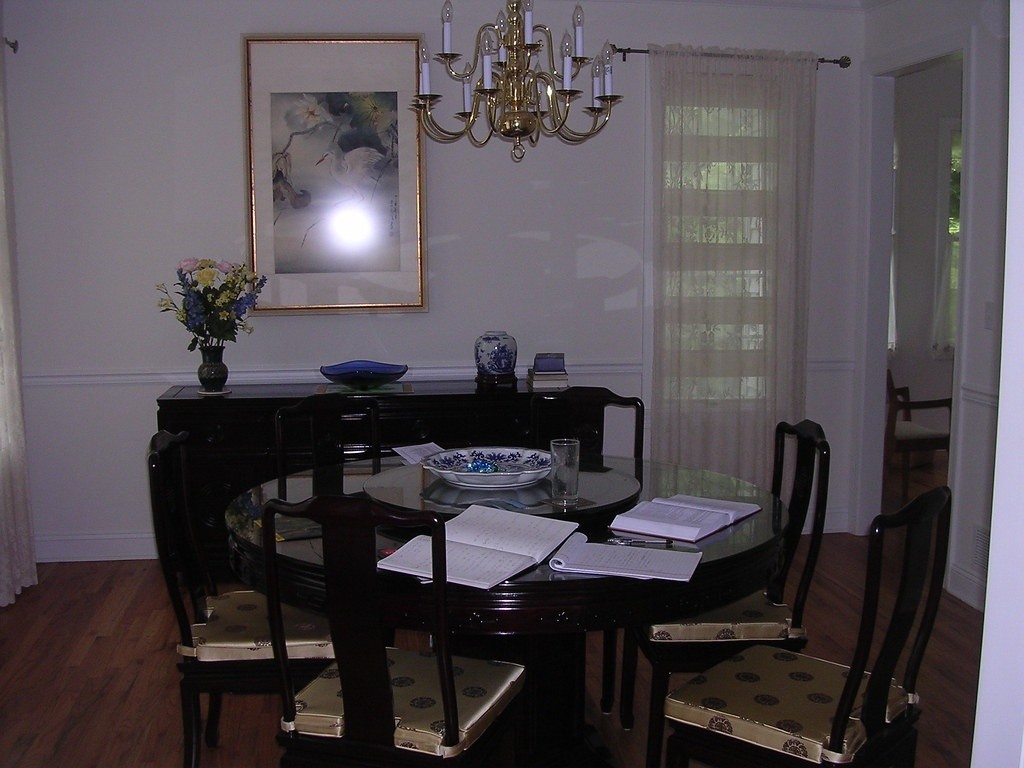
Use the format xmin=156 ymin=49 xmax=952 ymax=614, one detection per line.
xmin=474 ymin=331 xmax=518 ymax=373
xmin=198 ymin=346 xmax=229 ymax=392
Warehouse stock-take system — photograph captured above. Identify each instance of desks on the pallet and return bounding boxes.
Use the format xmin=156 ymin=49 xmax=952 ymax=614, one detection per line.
xmin=223 ymin=456 xmax=793 ymax=768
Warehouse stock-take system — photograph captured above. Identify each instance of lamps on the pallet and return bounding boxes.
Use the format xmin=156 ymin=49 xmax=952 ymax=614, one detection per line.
xmin=409 ymin=0 xmax=623 ymax=160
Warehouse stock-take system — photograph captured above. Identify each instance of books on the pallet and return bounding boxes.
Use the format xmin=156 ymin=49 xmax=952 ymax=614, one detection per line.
xmin=377 ymin=549 xmax=434 ymax=585
xmin=549 ymin=532 xmax=703 ymax=582
xmin=377 ymin=505 xmax=579 ymax=589
xmin=608 ymin=494 xmax=762 ymax=544
xmin=526 ymin=353 xmax=569 ymax=393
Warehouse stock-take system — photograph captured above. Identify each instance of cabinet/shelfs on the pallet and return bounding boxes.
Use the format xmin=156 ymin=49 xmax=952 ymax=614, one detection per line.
xmin=156 ymin=383 xmax=572 ymax=586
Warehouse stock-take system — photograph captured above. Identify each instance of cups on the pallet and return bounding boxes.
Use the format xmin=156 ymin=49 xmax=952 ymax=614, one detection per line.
xmin=551 ymin=439 xmax=579 ymax=505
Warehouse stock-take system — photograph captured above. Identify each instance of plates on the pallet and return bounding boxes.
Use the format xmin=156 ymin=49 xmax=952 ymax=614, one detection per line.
xmin=419 ymin=447 xmax=565 ymax=488
xmin=320 ymin=360 xmax=408 ymax=391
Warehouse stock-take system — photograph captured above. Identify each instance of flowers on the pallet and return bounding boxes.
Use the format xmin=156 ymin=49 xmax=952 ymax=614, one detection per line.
xmin=154 ymin=256 xmax=268 ymax=353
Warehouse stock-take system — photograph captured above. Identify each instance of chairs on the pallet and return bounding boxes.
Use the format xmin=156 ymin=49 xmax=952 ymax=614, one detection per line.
xmin=273 ymin=392 xmax=381 ymax=476
xmin=884 ymin=367 xmax=953 ymax=503
xmin=664 ymin=485 xmax=952 ymax=768
xmin=623 ymin=418 xmax=831 ymax=768
xmin=149 ymin=431 xmax=336 ymax=768
xmin=261 ymin=491 xmax=531 ymax=768
xmin=532 ymin=387 xmax=645 ymax=717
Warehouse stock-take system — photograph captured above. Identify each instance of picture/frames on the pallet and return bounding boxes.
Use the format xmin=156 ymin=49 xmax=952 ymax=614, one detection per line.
xmin=240 ymin=31 xmax=431 ymax=318
